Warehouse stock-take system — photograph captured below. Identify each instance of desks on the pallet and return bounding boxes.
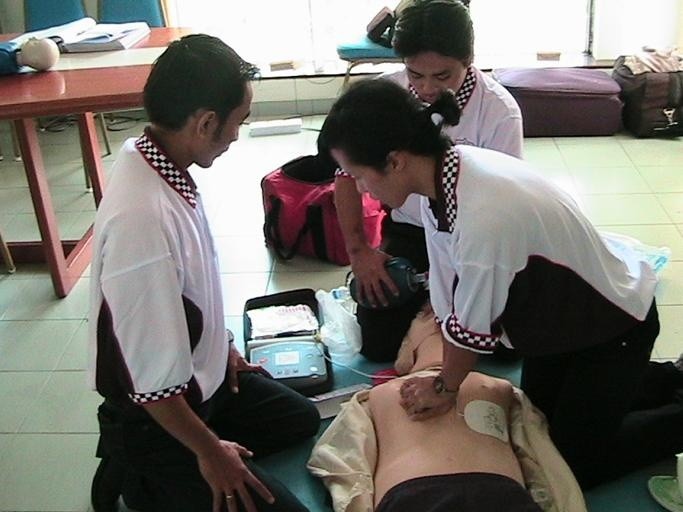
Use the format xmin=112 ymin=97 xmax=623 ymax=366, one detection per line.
xmin=0 ymin=22 xmax=193 ymax=302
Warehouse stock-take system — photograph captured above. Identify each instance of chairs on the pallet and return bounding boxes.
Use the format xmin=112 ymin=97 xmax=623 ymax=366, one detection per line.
xmin=0 ymin=2 xmax=185 ymax=135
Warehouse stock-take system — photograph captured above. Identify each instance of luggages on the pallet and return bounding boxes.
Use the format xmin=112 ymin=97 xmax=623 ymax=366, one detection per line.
xmin=490 ymin=66 xmax=625 ymax=138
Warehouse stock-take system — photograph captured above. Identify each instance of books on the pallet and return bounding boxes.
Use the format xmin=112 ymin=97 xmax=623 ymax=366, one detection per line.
xmin=9 ymin=16 xmax=152 ymax=53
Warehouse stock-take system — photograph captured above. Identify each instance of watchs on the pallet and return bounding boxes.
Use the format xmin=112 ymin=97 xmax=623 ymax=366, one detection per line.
xmin=432 ymin=375 xmax=461 ymax=396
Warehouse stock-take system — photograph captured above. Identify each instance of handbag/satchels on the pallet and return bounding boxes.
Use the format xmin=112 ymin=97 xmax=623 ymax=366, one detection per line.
xmin=259 ymin=154 xmax=389 ymax=265
xmin=612 ymin=52 xmax=683 ymax=136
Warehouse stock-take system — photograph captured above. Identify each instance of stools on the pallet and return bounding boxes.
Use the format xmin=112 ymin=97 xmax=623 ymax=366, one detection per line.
xmin=332 ymin=0 xmax=468 ymax=145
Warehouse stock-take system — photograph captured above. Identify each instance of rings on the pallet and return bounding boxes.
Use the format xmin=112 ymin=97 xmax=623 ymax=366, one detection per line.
xmin=225 ymin=494 xmax=235 ymax=499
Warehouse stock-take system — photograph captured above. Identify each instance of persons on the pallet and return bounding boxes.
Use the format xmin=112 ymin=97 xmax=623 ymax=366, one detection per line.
xmin=89 ymin=33 xmax=327 ymax=512
xmin=304 ymin=292 xmax=593 ymax=511
xmin=314 ymin=79 xmax=683 ymax=490
xmin=328 ymin=2 xmax=527 ymax=369
xmin=0 ymin=37 xmax=61 ymax=80
